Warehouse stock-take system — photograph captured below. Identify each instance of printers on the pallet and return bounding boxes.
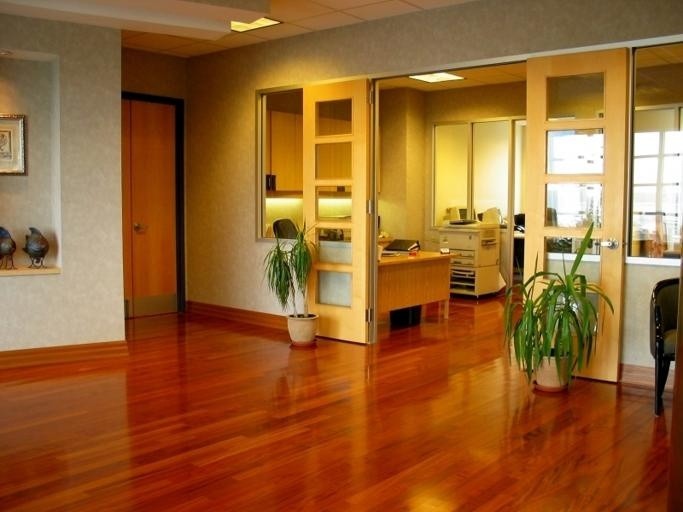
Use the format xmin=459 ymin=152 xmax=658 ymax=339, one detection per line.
xmin=438 ymin=219 xmax=501 ymax=268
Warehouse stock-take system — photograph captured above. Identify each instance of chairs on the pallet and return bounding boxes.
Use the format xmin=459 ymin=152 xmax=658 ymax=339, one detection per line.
xmin=272 ymin=218 xmax=297 ymax=239
xmin=647 ymin=276 xmax=679 ymax=417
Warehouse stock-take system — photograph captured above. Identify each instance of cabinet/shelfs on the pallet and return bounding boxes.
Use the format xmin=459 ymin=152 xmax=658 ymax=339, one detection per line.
xmin=265 ymin=110 xmax=351 ymax=194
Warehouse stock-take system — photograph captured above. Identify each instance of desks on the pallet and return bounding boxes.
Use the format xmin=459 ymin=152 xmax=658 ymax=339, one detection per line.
xmin=376 ymin=251 xmax=460 ymax=331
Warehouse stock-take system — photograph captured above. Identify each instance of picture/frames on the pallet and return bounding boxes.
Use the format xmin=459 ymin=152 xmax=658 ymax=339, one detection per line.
xmin=0 ymin=114 xmax=26 ymax=177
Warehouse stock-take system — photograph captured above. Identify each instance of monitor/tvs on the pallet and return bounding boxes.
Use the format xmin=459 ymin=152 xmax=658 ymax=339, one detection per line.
xmin=450 ymin=208 xmax=477 ymax=224
xmin=481 ymin=207 xmax=503 ymax=224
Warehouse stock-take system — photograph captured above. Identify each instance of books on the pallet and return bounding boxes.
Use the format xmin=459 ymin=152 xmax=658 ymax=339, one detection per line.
xmin=384 ymin=238 xmax=418 ymax=251
xmin=381 ymin=246 xmax=419 ymax=254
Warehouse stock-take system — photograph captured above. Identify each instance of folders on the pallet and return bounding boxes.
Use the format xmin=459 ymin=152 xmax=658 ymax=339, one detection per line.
xmin=384 ymin=240 xmax=420 ymax=251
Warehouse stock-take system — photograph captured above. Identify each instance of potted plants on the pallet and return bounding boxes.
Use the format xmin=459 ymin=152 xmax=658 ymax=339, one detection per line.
xmin=262 ymin=217 xmax=319 ymax=349
xmin=500 ymin=230 xmax=615 ymax=389
